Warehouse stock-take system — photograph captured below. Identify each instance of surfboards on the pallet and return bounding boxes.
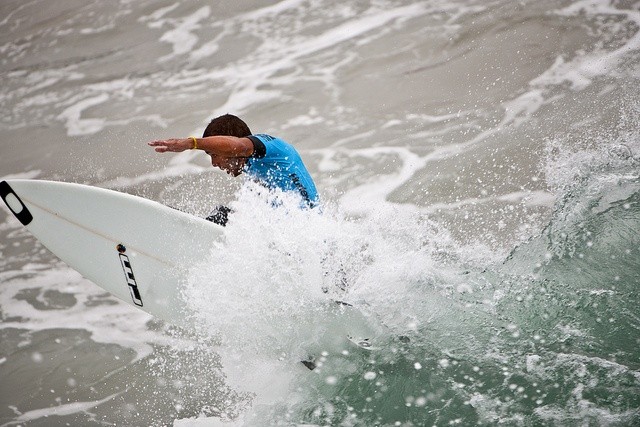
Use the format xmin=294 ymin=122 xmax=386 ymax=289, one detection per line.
xmin=0 ymin=177 xmax=386 ymax=366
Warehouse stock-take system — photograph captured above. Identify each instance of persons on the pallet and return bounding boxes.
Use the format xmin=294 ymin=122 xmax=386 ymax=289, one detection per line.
xmin=147 ymin=113 xmax=321 ymax=227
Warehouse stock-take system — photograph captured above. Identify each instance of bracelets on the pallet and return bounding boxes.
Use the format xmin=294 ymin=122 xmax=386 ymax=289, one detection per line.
xmin=188 ymin=137 xmax=197 ymax=150
